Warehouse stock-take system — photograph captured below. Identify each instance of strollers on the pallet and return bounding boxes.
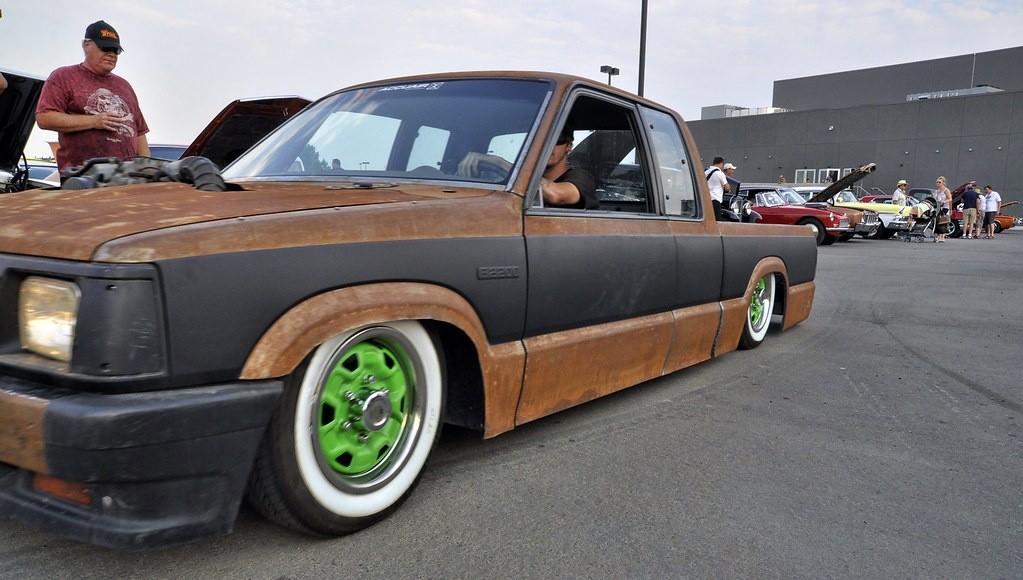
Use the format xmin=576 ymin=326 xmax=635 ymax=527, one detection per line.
xmin=897 ymin=197 xmax=944 ymax=243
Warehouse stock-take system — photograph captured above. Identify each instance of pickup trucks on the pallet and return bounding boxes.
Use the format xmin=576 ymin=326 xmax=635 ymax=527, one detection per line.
xmin=0 ymin=69 xmax=819 ymax=550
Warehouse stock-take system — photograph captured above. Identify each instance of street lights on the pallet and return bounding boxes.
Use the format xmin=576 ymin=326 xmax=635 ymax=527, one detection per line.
xmin=600 ymin=65 xmax=620 ymax=87
xmin=362 ymin=161 xmax=370 ymax=169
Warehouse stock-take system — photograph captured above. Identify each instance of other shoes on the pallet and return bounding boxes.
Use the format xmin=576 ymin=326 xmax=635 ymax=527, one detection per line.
xmin=960 ymin=235 xmax=993 ymax=239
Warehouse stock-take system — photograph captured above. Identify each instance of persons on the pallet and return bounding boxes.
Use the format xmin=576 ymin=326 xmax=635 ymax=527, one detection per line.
xmin=704 ymin=157 xmax=730 ymax=221
xmin=961 ymin=184 xmax=980 ymax=239
xmin=456 ymin=114 xmax=599 ymax=212
xmin=723 ymin=163 xmax=737 ymax=177
xmin=891 ymin=180 xmax=908 ymax=206
xmin=35 ymin=20 xmax=152 ymax=188
xmin=984 ymin=184 xmax=1001 ymax=240
xmin=332 ymin=159 xmax=344 ymax=171
xmin=933 ymin=176 xmax=952 ymax=242
xmin=973 ymin=188 xmax=986 ymax=238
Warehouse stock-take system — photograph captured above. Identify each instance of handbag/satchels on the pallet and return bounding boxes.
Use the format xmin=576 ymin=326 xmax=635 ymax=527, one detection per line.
xmin=939 ymin=210 xmax=950 ymax=224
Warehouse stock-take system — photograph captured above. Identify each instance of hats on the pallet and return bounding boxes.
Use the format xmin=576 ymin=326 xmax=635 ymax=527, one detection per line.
xmin=975 ymin=188 xmax=981 ymax=194
xmin=723 ymin=163 xmax=737 ymax=169
xmin=897 ymin=179 xmax=907 ymax=187
xmin=84 ymin=19 xmax=123 ymax=50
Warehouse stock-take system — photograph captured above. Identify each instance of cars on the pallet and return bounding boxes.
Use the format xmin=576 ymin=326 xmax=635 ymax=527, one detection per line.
xmin=0 ymin=70 xmax=319 ymax=197
xmin=601 ymin=159 xmax=1020 ymax=247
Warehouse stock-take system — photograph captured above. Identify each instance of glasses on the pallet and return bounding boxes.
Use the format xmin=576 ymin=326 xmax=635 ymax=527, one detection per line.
xmin=97 ymin=44 xmax=122 ymax=54
xmin=902 ymin=184 xmax=906 ymax=187
xmin=936 ymin=181 xmax=942 ymax=184
xmin=557 ymin=135 xmax=571 ymax=145
